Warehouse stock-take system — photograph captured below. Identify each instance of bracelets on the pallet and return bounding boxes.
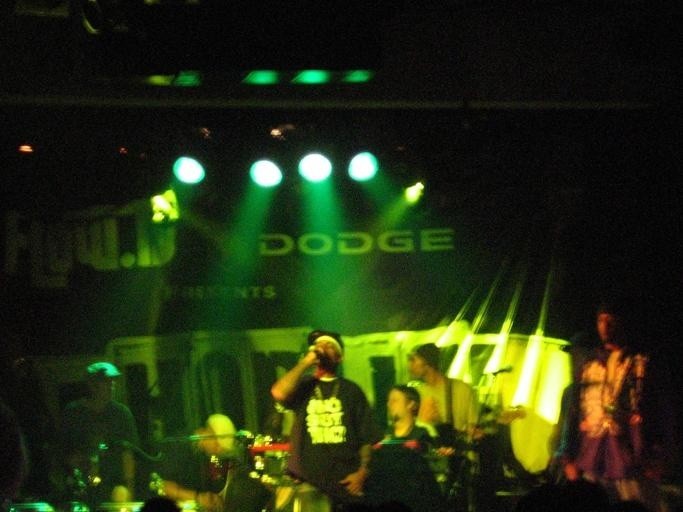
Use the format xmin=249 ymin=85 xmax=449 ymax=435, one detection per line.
xmin=362 ymin=460 xmax=368 ymax=471
xmin=195 ymin=489 xmax=198 ymax=501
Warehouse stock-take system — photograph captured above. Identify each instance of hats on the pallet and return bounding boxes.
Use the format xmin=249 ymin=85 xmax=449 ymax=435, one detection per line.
xmin=84 ymin=363 xmax=123 ymax=378
xmin=417 ymin=344 xmax=439 ymax=369
xmin=309 ymin=331 xmax=344 ymax=355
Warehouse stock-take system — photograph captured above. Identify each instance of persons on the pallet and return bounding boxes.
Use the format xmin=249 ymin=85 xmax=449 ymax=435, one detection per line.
xmin=368 ymin=386 xmax=457 ymax=456
xmin=271 ymin=331 xmax=371 ymax=508
xmin=516 ymin=478 xmax=612 ymax=511
xmin=547 ymin=307 xmax=683 ymax=512
xmin=164 ymin=414 xmax=237 ymax=511
xmin=50 ymin=363 xmax=137 ymax=503
xmin=360 ymin=444 xmax=446 ymax=512
xmin=139 ymin=495 xmax=182 ymax=511
xmin=406 ymin=342 xmax=484 ymax=511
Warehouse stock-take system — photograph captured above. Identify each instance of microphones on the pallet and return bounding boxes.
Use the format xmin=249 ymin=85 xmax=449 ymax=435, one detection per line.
xmin=237 ymin=429 xmax=255 ymax=445
xmin=313 ymin=348 xmax=328 ymax=360
xmin=492 ymin=365 xmax=512 ymax=375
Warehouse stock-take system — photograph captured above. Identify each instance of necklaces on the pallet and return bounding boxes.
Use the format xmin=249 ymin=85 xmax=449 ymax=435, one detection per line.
xmin=315 ymin=379 xmax=340 ymax=422
